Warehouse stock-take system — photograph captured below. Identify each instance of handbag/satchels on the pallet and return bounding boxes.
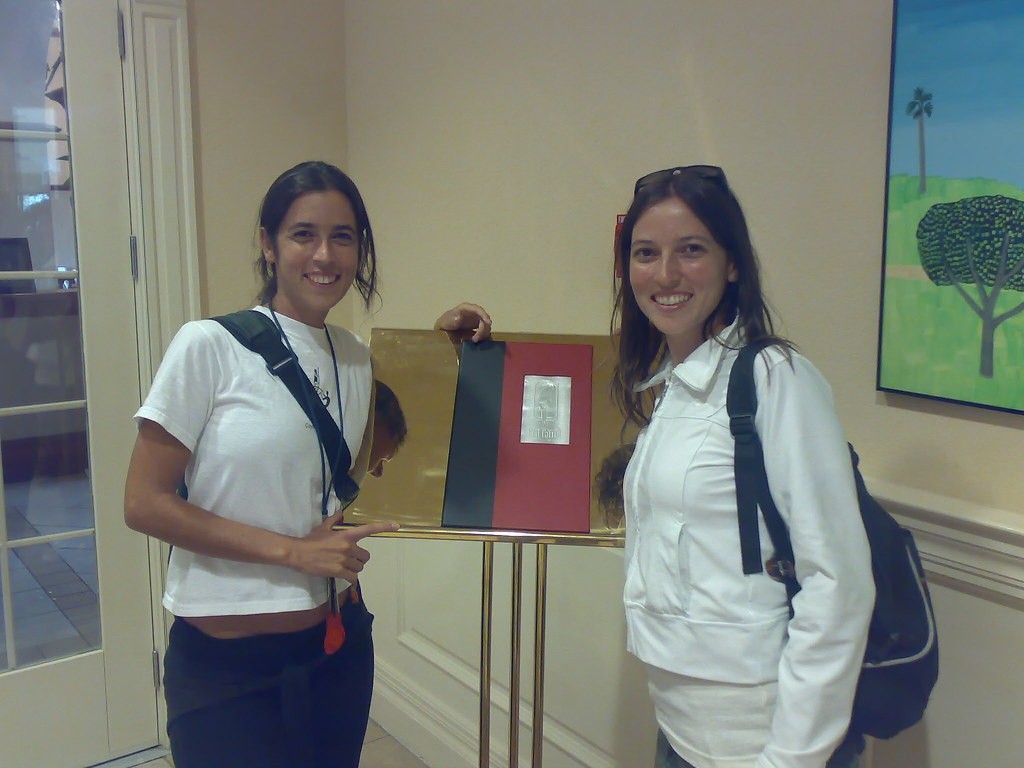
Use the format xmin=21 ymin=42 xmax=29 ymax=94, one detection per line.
xmin=726 ymin=337 xmax=939 ymax=739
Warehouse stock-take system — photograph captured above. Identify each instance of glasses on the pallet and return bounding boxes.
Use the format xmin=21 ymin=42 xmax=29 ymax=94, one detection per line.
xmin=634 ymin=165 xmax=728 ymax=195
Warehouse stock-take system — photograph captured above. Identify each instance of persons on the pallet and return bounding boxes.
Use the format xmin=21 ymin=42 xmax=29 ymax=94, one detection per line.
xmin=609 ymin=166 xmax=875 ymax=767
xmin=123 ymin=160 xmax=492 ymax=767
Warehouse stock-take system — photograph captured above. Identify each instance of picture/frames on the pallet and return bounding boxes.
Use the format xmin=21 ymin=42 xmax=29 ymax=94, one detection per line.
xmin=876 ymin=0 xmax=1024 ymax=415
